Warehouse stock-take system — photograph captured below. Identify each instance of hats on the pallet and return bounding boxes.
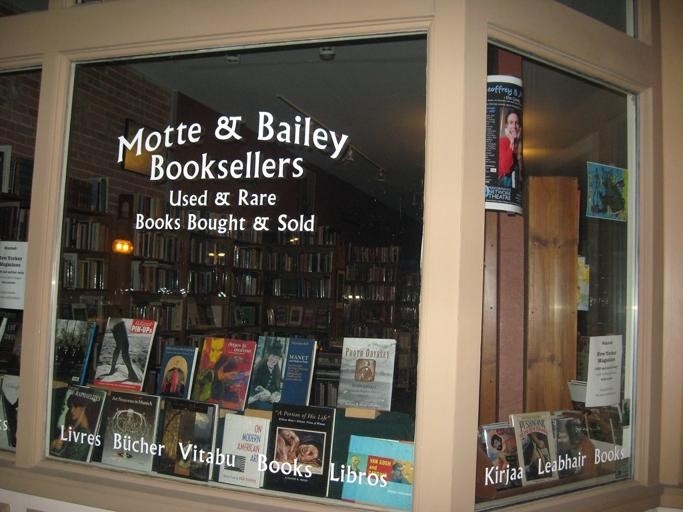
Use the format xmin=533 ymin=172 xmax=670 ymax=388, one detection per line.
xmin=265 ymin=342 xmax=283 ymax=357
xmin=67 ymin=387 xmax=111 ymax=409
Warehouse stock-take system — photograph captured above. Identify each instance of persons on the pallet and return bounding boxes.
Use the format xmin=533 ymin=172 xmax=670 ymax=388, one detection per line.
xmin=109 ymin=318 xmax=142 ymax=382
xmin=497 ymin=109 xmax=522 ymax=184
xmin=169 ymin=367 xmax=181 ymax=395
xmin=525 ymin=429 xmax=551 ymax=475
xmin=51 ymin=388 xmax=91 ymax=460
xmin=161 ymin=355 xmax=187 ymax=395
xmin=191 ymin=353 xmax=240 ymax=402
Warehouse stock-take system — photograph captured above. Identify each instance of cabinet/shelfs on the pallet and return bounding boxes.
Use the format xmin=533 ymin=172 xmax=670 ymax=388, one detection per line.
xmin=344 ymin=243 xmax=398 ymax=340
xmin=117 ymin=193 xmax=184 ymax=395
xmin=185 ymin=208 xmax=264 ymax=348
xmin=263 ymin=221 xmax=350 ymax=407
xmin=397 ymin=263 xmax=421 ymax=392
xmin=526 ymin=174 xmax=597 ymax=482
xmin=475 ymin=44 xmax=527 ymax=507
xmin=0 ymin=145 xmax=117 ymax=375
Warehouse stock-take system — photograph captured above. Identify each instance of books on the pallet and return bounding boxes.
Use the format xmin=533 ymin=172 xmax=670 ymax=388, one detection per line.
xmin=118 ymin=193 xmax=178 ymax=220
xmin=247 ymin=336 xmax=317 ymax=406
xmin=53 ymin=318 xmax=97 ymax=386
xmin=341 ymin=434 xmax=416 ymax=512
xmin=262 ymin=402 xmax=336 ymax=498
xmin=93 ymin=316 xmax=158 ymax=393
xmin=234 ymin=306 xmax=255 ymax=326
xmin=59 ymin=294 xmax=103 ymax=333
xmin=336 ymin=338 xmax=397 ymax=412
xmin=190 ymin=237 xmax=225 ymax=267
xmin=346 ymin=263 xmax=398 ymax=284
xmin=0 ymin=144 xmax=33 ymax=210
xmin=187 ymin=303 xmax=222 ymax=330
xmin=267 ymin=305 xmax=331 ymax=329
xmin=156 ymin=345 xmax=199 ymax=401
xmin=191 ymin=336 xmax=257 ymax=412
xmin=209 ymin=212 xmax=263 ymax=244
xmin=65 ymin=175 xmax=110 ymax=215
xmin=151 ymin=397 xmax=219 ymax=482
xmin=128 ymin=298 xmax=184 ymax=331
xmin=481 ymin=426 xmax=523 ymax=492
xmin=233 ymin=245 xmax=333 ymax=273
xmin=348 ymin=247 xmax=399 ymax=264
xmin=0 ymin=308 xmax=24 ymax=376
xmin=133 ymin=224 xmax=179 ymax=263
xmin=0 ymin=374 xmax=21 ymax=453
xmin=278 ymin=226 xmax=346 ymax=245
xmin=188 ymin=271 xmax=224 ymax=296
xmin=236 ymin=274 xmax=332 ymax=299
xmin=247 ymin=354 xmax=282 ymax=404
xmin=343 ymin=304 xmax=397 ymax=339
xmin=510 ymin=411 xmax=560 ymax=487
xmin=341 ymin=284 xmax=396 ymax=302
xmin=100 ymin=388 xmax=161 ymax=474
xmin=488 ymin=432 xmax=504 ymax=452
xmin=485 ymin=74 xmax=525 ymax=215
xmin=62 ymin=216 xmax=112 ymax=254
xmin=0 ymin=201 xmax=26 ymax=241
xmin=396 ymin=271 xmax=420 ymax=337
xmin=132 ymin=260 xmax=178 ymax=294
xmin=49 ymin=382 xmax=107 ymax=465
xmin=62 ymin=253 xmax=106 ymax=290
xmin=218 ymin=413 xmax=270 ymax=489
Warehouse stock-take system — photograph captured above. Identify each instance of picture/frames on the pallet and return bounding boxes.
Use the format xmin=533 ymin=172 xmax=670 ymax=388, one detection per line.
xmin=585 ymin=160 xmax=629 ymax=223
